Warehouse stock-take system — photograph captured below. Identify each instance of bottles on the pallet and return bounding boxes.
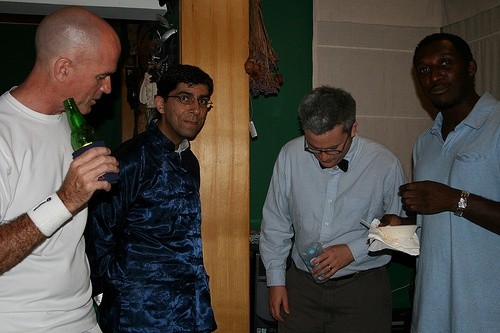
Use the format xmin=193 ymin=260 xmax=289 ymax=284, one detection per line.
xmin=63 ymin=98 xmax=117 ymax=190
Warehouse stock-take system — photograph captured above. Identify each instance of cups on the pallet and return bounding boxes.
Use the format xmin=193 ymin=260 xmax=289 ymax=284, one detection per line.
xmin=299 ymin=242 xmax=330 ymax=284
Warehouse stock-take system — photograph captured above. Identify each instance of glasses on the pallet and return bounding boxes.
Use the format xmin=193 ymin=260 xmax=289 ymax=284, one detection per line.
xmin=165 ymin=95 xmax=213 ymax=110
xmin=304 ymin=134 xmax=349 ymax=155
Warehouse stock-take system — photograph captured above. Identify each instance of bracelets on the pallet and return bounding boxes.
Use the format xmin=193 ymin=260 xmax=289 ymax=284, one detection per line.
xmin=457 ymin=190 xmax=471 ymax=217
xmin=28 ymin=193 xmax=73 ymax=238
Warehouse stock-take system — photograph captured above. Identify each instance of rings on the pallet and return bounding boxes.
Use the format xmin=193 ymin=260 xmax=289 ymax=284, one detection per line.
xmin=327 ymin=264 xmax=332 ymax=270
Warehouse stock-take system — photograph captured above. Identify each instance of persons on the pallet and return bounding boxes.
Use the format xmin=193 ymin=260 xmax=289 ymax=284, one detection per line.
xmin=379 ymin=32 xmax=500 ymax=333
xmin=259 ymin=86 xmax=410 ymax=333
xmin=84 ymin=63 xmax=219 ymax=333
xmin=0 ymin=6 xmax=122 ymax=333
